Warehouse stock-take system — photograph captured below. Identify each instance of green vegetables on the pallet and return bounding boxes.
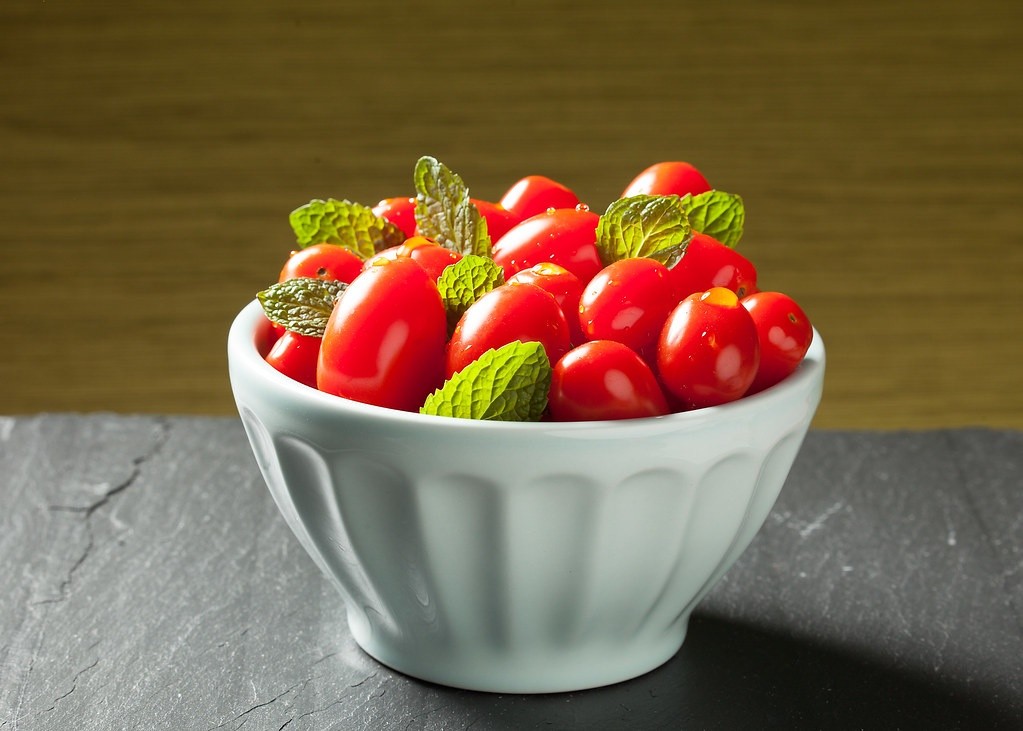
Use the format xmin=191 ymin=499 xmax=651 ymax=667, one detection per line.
xmin=256 ymin=155 xmax=745 ymax=421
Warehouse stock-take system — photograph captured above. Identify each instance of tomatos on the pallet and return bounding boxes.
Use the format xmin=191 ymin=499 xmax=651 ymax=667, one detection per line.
xmin=267 ymin=161 xmax=813 ymax=419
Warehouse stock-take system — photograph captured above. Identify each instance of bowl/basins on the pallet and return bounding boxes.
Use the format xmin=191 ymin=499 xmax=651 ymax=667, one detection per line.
xmin=227 ymin=297 xmax=824 ymax=694
